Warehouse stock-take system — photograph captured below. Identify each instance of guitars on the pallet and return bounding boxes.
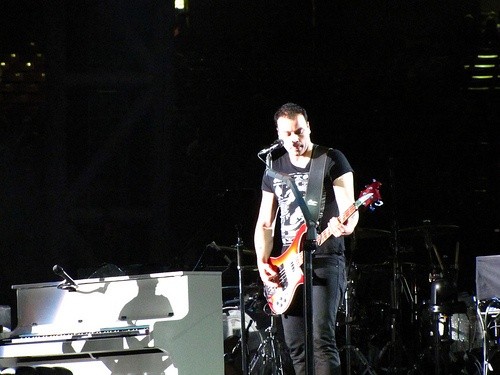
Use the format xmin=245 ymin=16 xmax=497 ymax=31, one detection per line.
xmin=260 ymin=179 xmax=386 ymax=317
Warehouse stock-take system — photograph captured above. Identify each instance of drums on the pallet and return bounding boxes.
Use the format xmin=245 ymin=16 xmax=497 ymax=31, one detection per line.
xmin=446 ymin=311 xmax=490 ymax=352
xmin=223 ymin=299 xmax=293 ymax=375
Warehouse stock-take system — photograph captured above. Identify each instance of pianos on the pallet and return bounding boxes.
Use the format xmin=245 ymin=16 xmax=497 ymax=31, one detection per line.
xmin=0 ymin=271 xmax=225 ymax=375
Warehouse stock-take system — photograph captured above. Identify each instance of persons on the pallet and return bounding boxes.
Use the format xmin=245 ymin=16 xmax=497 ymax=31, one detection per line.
xmin=253 ymin=103 xmax=360 ymax=375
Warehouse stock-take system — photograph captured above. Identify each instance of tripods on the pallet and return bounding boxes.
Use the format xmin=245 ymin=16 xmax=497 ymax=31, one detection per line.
xmin=338 ymin=266 xmax=376 ymax=375
xmin=248 ymin=309 xmax=288 ymax=375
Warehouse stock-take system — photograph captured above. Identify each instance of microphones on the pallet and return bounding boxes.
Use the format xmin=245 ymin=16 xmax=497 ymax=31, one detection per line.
xmin=52 ymin=265 xmax=77 ymax=287
xmin=260 ymin=139 xmax=284 ymax=154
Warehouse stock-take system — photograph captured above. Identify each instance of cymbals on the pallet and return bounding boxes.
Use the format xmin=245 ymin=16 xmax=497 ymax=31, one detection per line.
xmin=348 ymin=226 xmax=392 ymax=242
xmin=397 ymin=223 xmax=461 ymax=235
